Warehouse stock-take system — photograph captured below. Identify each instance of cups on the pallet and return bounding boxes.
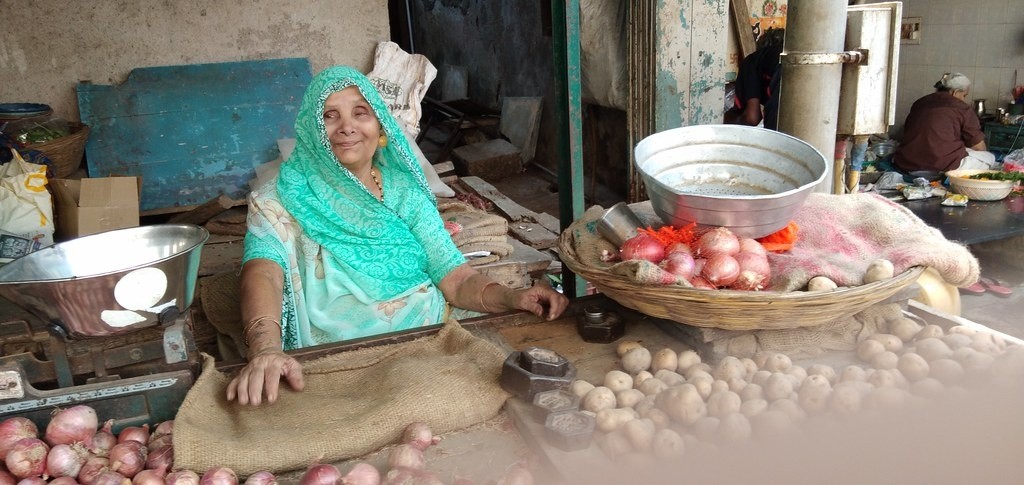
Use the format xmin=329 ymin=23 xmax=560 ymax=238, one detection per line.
xmin=597 ymin=202 xmax=646 ymax=250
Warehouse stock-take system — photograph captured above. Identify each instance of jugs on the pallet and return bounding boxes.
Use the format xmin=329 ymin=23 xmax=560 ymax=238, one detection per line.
xmin=974 ymin=98 xmax=986 ymax=117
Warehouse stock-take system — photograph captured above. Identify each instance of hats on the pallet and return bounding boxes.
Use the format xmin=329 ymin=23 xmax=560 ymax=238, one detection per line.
xmin=941 ymin=73 xmax=969 ymax=89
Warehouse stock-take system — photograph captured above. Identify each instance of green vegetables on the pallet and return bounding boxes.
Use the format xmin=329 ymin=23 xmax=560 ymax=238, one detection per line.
xmin=970 ymin=169 xmax=1024 ymax=197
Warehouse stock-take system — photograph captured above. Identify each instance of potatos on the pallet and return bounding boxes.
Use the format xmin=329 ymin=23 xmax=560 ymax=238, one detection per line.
xmin=865 ymin=259 xmax=896 ymax=284
xmin=809 ymin=278 xmax=839 ymax=296
xmin=571 ymin=318 xmax=1024 ymax=466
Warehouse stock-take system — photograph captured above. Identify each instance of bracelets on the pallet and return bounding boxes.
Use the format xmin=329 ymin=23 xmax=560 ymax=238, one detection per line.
xmin=479 ymin=280 xmax=510 ymax=313
xmin=241 ymin=313 xmax=286 ymax=349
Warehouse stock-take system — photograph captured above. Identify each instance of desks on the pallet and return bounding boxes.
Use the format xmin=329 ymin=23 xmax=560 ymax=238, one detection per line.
xmin=218 ymin=294 xmax=1024 ymax=485
xmin=881 ymin=187 xmax=1024 ymax=245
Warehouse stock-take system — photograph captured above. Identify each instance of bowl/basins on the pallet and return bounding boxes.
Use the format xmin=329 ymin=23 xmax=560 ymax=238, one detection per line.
xmin=860 ymin=166 xmax=885 ymax=183
xmin=872 ymin=143 xmax=897 ymax=157
xmin=0 ymin=224 xmax=209 ymax=345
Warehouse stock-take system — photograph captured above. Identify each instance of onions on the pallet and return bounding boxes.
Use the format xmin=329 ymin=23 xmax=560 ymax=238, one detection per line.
xmin=299 ymin=422 xmax=532 ymax=485
xmin=620 ymin=228 xmax=772 ymax=290
xmin=0 ymin=405 xmax=277 ymax=485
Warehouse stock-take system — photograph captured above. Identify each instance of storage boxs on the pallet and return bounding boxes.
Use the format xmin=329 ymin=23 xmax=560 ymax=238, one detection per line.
xmin=459 ymin=95 xmax=544 ymax=181
xmin=46 ymin=172 xmax=141 ymax=243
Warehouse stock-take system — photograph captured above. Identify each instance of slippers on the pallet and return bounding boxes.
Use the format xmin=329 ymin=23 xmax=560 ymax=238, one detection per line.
xmin=957 ymin=282 xmax=986 ymax=295
xmin=980 ymin=276 xmax=1012 ymax=297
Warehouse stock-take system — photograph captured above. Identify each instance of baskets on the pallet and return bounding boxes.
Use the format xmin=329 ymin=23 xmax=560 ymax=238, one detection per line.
xmin=946 ymin=169 xmax=1015 ymax=200
xmin=7 ymin=121 xmax=90 ymax=177
xmin=557 ymin=200 xmax=928 ymax=330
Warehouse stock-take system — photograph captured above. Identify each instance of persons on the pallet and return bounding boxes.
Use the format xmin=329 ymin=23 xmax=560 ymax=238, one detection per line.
xmin=225 ymin=65 xmax=568 ymax=404
xmin=895 ymin=71 xmax=995 ymax=177
xmin=730 ymin=35 xmax=784 ymax=133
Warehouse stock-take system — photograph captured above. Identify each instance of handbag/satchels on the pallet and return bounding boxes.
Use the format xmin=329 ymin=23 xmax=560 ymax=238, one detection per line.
xmin=0 ymin=148 xmax=55 ymax=257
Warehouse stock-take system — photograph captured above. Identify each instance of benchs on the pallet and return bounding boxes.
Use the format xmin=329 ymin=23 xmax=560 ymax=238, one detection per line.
xmin=415 ymin=91 xmax=498 ymax=164
